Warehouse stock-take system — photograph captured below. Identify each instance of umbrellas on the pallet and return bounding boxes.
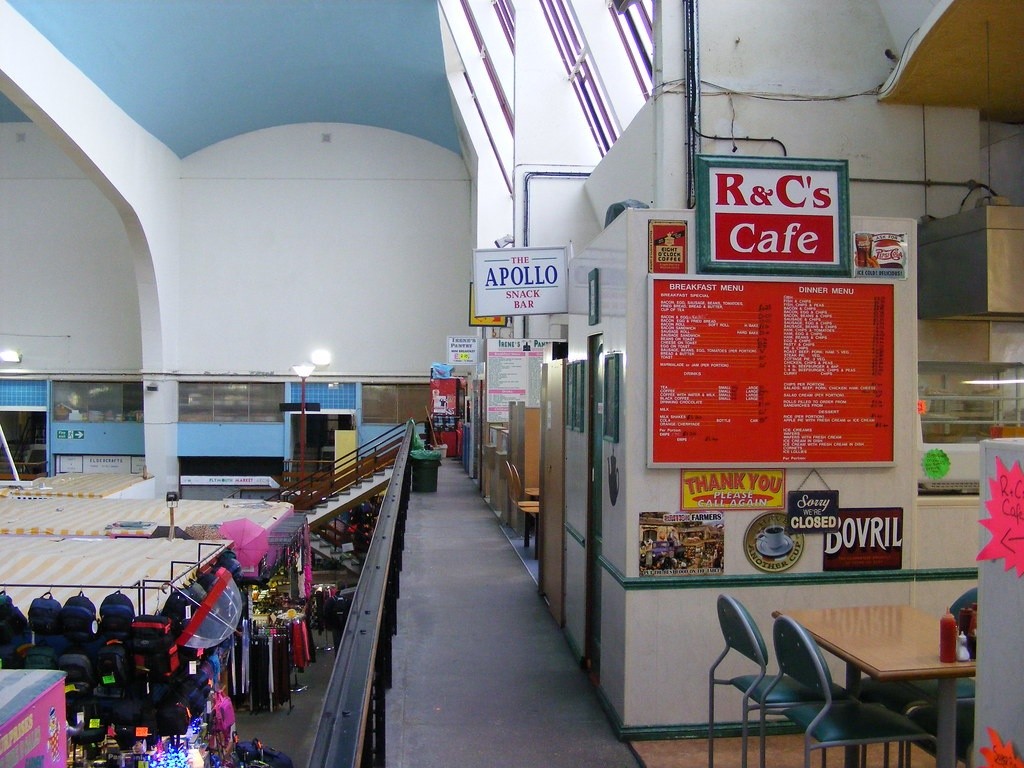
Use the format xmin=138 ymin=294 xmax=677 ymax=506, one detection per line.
xmin=161 ymin=571 xmax=243 ymax=648
xmin=151 ymin=517 xmax=269 ymax=567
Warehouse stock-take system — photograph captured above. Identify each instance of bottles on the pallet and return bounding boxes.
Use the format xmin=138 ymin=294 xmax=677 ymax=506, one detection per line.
xmin=939 ymin=601 xmax=977 ymax=662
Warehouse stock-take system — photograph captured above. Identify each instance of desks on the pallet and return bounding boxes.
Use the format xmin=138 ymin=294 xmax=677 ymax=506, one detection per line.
xmin=770 ymin=603 xmax=977 ymax=768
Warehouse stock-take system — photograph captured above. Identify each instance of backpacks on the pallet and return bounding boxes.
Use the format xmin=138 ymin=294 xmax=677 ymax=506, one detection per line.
xmin=331 ymin=595 xmax=347 ymax=630
xmin=0 ymin=547 xmax=245 ymax=759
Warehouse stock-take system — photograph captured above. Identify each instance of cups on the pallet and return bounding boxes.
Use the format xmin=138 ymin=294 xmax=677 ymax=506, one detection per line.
xmin=758 ymin=526 xmax=784 ymax=548
xmin=856 ymin=232 xmax=871 ymax=266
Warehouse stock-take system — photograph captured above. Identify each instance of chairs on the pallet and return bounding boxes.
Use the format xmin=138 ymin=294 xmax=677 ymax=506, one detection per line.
xmin=505 ymin=460 xmax=539 ymax=559
xmin=708 ymin=588 xmax=978 ymax=768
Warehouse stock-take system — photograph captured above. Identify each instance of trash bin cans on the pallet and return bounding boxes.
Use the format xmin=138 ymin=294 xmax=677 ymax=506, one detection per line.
xmin=410 ymin=450 xmax=442 ymax=493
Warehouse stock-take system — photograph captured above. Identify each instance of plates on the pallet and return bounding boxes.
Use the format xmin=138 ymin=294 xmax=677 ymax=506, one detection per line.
xmin=756 ymin=533 xmax=793 ymax=556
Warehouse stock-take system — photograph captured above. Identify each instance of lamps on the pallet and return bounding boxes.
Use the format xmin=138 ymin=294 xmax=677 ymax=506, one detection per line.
xmin=311 ymin=349 xmax=331 ymax=365
xmin=0 ymin=351 xmax=23 ymax=363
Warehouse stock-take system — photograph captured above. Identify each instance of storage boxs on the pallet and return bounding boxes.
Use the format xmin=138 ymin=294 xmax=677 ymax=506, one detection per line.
xmin=430 ymin=415 xmax=461 ymax=430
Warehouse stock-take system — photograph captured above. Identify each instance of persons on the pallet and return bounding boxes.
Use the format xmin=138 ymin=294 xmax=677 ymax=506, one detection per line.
xmin=253 ymin=586 xmax=349 ymax=657
xmin=343 ymin=495 xmax=382 ymax=560
xmin=640 ymin=529 xmax=723 ymax=570
